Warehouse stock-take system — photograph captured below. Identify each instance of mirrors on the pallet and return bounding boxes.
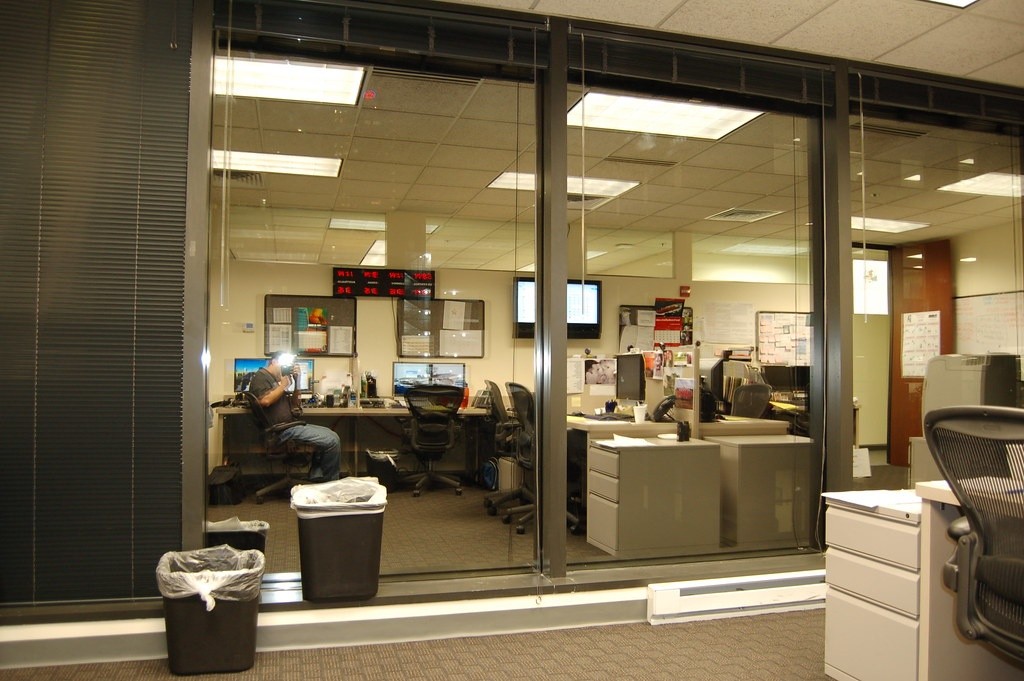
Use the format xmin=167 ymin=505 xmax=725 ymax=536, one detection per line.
xmin=202 ymin=0 xmax=1024 ymax=592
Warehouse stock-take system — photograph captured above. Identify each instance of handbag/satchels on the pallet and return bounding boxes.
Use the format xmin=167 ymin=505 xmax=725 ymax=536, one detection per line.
xmin=477 ymin=456 xmax=498 ymax=491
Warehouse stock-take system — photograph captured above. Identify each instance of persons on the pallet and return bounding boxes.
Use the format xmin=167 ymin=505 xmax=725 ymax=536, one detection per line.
xmin=249 ymin=351 xmax=341 ymax=482
xmin=585 ymin=360 xmax=617 ymax=385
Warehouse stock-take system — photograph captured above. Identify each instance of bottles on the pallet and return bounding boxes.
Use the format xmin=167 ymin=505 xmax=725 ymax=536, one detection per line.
xmin=683 ymin=421 xmax=690 ymax=441
xmin=341 ymin=384 xmax=345 ymax=393
xmin=677 ymin=422 xmax=684 ymax=442
xmin=334 ymin=391 xmax=340 ymax=407
xmin=345 ymin=373 xmax=351 ymax=386
xmin=326 ymin=389 xmax=334 ymax=408
xmin=341 ymin=395 xmax=348 ymax=408
xmin=461 ymin=383 xmax=469 ymax=410
xmin=350 ymin=394 xmax=356 ymax=408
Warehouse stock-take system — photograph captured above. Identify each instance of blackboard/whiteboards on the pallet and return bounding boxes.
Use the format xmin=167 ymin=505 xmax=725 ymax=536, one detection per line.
xmin=951 ymin=290 xmax=1024 ymax=356
xmin=756 ymin=312 xmax=810 ymax=366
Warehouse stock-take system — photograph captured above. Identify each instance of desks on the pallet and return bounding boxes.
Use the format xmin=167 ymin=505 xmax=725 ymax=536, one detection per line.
xmin=915 ymin=476 xmax=1024 ymax=681
xmin=566 ymin=416 xmax=678 ymax=440
xmin=215 ymin=406 xmax=492 ymax=489
xmin=699 ymin=414 xmax=791 ymax=440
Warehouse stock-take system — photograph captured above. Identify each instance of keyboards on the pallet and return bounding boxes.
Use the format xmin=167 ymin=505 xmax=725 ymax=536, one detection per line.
xmin=597 ymin=412 xmax=633 ymax=420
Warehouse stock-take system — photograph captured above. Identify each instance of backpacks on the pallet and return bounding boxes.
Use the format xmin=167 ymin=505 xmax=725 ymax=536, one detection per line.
xmin=207 ymin=461 xmax=241 ymax=507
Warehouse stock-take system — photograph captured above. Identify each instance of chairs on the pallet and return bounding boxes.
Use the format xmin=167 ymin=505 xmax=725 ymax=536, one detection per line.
xmin=793 ymin=383 xmax=810 ymax=437
xmin=501 ymin=382 xmax=583 ymax=534
xmin=393 ymin=382 xmax=465 ymax=497
xmin=730 ymin=383 xmax=772 ymax=418
xmin=242 ymin=391 xmax=318 ymax=505
xmin=923 ymin=405 xmax=1024 ymax=664
xmin=483 ymin=379 xmax=523 ymax=517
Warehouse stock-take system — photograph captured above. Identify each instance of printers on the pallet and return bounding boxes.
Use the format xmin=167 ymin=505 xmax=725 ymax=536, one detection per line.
xmin=910 ymin=353 xmax=1022 ymax=491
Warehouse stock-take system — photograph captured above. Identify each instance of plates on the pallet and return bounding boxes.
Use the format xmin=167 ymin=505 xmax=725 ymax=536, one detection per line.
xmin=658 ymin=434 xmax=677 ymax=439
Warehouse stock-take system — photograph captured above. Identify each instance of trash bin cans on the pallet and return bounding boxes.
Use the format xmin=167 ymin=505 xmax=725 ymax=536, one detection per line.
xmin=155 ymin=546 xmax=267 ymax=676
xmin=290 ymin=475 xmax=388 ymax=602
xmin=366 ymin=448 xmax=400 ymax=492
xmin=206 ymin=516 xmax=271 ymax=554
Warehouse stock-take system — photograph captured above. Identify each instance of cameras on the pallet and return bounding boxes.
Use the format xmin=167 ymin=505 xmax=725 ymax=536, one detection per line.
xmin=280 ymin=364 xmax=294 ymax=377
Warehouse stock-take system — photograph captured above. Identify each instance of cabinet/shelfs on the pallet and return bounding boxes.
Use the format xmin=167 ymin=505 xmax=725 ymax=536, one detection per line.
xmin=586 ymin=435 xmax=719 ymax=556
xmin=820 ymin=488 xmax=922 ymax=681
xmin=703 ymin=434 xmax=813 ymax=547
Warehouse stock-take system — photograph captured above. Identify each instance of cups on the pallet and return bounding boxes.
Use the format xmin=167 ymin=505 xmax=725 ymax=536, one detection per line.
xmin=633 ymin=406 xmax=646 ymax=423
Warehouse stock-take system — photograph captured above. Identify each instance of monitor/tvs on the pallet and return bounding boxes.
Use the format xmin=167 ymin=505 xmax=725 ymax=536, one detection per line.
xmin=233 ymin=358 xmax=314 ymax=395
xmin=617 ymin=354 xmax=647 ymax=405
xmin=512 ymin=276 xmax=602 ymax=340
xmin=760 ymin=365 xmax=812 ymax=393
xmin=392 ymin=362 xmax=466 ymax=396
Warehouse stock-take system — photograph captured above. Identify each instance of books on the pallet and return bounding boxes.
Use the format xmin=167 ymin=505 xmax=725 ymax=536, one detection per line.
xmin=722 ymin=374 xmax=750 ymax=404
